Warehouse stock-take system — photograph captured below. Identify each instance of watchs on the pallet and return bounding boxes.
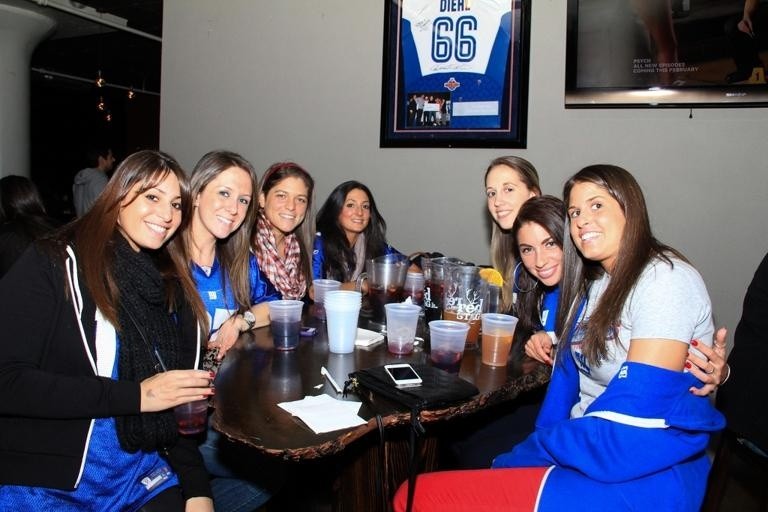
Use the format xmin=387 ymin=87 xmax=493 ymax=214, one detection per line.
xmin=238 ymin=305 xmax=258 ymax=332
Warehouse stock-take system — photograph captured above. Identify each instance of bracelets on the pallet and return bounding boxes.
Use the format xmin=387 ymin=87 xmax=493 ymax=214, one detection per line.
xmin=719 ymin=362 xmax=730 ymax=386
xmin=354 ymin=270 xmax=370 ymax=294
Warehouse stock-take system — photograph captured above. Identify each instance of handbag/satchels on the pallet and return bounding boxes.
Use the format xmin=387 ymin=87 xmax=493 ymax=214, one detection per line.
xmin=342 ymin=363 xmax=479 ymax=512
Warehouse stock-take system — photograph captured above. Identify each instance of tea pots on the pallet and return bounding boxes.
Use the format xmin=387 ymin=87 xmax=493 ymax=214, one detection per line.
xmin=355 ymin=254 xmax=410 ymax=323
xmin=423 ymin=257 xmax=465 ymax=327
xmin=442 ymin=264 xmax=490 ymax=352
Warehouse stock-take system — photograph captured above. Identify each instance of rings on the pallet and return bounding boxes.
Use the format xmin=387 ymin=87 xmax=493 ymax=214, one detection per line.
xmin=706 ymin=365 xmax=715 ymax=376
xmin=713 ymin=339 xmax=728 ymax=349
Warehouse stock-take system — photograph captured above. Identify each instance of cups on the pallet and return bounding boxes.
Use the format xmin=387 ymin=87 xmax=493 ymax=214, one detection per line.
xmin=481 ymin=312 xmax=519 ymax=367
xmin=404 ymin=273 xmax=425 ymax=305
xmin=268 ymin=300 xmax=304 ymax=349
xmin=312 ymin=279 xmax=341 ymax=319
xmin=385 ymin=302 xmax=422 ymax=355
xmin=323 ymin=290 xmax=362 ymax=354
xmin=427 ymin=320 xmax=470 ymax=377
xmin=176 ymin=401 xmax=208 ymax=435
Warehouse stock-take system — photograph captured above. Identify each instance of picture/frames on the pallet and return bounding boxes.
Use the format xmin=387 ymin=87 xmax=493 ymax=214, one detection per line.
xmin=379 ymin=0 xmax=531 ymax=151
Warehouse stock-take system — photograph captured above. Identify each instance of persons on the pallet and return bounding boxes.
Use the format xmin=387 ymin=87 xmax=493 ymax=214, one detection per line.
xmin=312 ymin=180 xmax=411 ymax=299
xmin=724 ymin=0 xmax=767 ymax=83
xmin=510 ymin=196 xmax=732 ymax=398
xmin=249 ymin=161 xmax=315 ymax=319
xmin=408 ymin=94 xmax=447 ymax=126
xmin=72 ymin=141 xmax=116 ymax=219
xmin=182 ymin=150 xmax=283 ymax=512
xmin=392 ymin=164 xmax=715 ymax=512
xmin=0 ymin=148 xmax=213 ymax=512
xmin=0 ymin=174 xmax=51 ymax=274
xmin=488 ymin=156 xmax=542 ymax=304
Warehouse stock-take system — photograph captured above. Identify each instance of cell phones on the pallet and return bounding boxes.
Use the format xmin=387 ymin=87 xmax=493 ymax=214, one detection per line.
xmin=384 ymin=363 xmax=423 ymax=386
